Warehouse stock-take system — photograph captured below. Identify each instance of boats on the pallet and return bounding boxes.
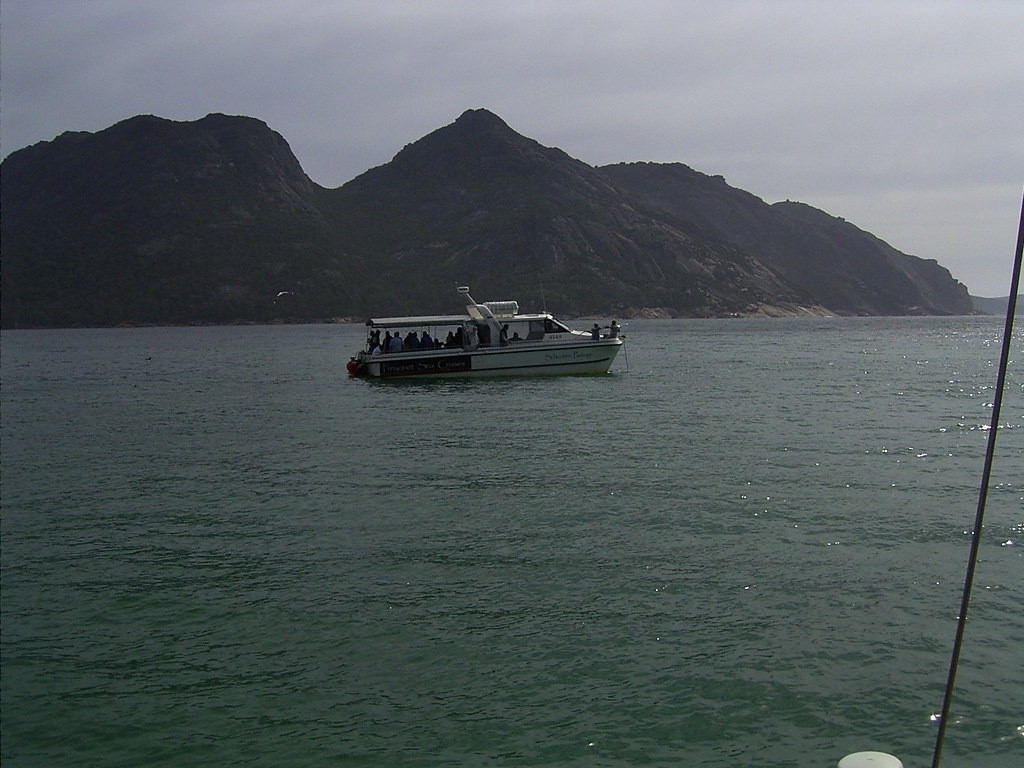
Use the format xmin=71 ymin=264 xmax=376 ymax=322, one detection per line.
xmin=348 ymin=284 xmax=629 ymax=384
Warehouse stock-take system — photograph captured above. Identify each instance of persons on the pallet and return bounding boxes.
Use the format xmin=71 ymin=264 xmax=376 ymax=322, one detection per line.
xmin=591 ymin=322 xmax=602 ymax=340
xmin=510 ymin=332 xmax=523 ymax=341
xmin=367 ymin=327 xmax=463 ymax=355
xmin=605 ymin=321 xmax=621 ymax=339
xmin=500 ymin=324 xmax=512 ymax=347
